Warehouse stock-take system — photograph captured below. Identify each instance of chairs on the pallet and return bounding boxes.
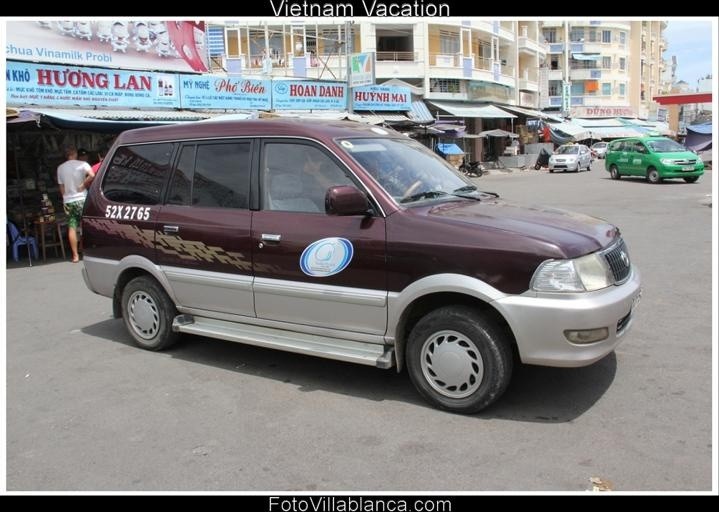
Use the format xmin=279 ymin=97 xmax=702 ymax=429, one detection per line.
xmin=271 ymin=173 xmax=321 ymax=214
xmin=8 ymin=222 xmax=40 ymax=262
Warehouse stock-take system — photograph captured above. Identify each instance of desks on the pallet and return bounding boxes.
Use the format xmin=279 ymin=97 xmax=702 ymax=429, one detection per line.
xmin=33 ymin=217 xmax=67 ymax=262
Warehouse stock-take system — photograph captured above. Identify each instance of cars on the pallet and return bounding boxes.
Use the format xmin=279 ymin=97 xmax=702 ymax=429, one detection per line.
xmin=548 ymin=141 xmax=593 ymax=173
xmin=590 ymin=141 xmax=609 ymax=159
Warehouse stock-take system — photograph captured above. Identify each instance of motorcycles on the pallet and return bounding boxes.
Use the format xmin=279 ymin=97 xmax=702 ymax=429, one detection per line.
xmin=458 ymin=156 xmax=483 ymax=177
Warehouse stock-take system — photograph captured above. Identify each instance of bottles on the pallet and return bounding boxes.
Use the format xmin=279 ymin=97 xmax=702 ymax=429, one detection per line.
xmin=40 ymin=199 xmax=56 ymax=222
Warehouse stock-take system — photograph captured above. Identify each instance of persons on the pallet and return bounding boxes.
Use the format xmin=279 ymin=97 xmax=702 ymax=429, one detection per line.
xmin=56 ymin=143 xmax=109 ymax=263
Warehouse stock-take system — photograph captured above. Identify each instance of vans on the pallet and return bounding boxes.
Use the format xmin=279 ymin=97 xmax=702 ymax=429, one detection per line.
xmin=604 ymin=136 xmax=704 ymax=183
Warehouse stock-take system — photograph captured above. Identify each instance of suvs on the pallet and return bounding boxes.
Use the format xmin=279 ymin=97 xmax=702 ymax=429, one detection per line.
xmin=77 ymin=114 xmax=642 ymax=414
